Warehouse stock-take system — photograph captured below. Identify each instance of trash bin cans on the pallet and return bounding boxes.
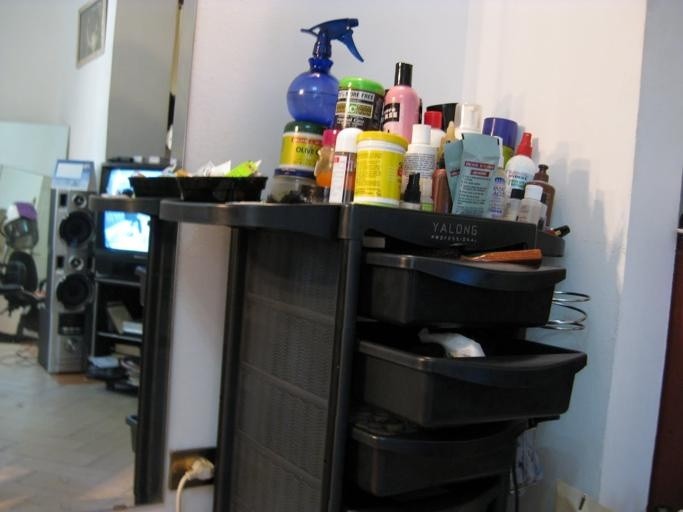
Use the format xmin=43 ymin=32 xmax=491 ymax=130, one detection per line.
xmin=127 ymin=414 xmax=137 ymax=454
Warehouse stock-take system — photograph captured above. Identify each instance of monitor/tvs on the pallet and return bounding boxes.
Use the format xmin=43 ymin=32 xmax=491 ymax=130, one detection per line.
xmin=92 ymin=161 xmax=170 ymax=283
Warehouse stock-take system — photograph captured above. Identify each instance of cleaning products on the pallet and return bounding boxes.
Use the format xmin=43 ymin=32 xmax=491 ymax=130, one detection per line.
xmin=287 ymin=16 xmax=363 ymax=125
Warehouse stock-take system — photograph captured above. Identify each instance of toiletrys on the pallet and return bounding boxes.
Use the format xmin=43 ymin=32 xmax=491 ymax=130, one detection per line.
xmin=448 ymin=117 xmax=570 ymax=237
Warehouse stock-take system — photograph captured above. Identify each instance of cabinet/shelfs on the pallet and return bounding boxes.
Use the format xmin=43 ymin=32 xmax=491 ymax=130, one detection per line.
xmin=86 ymin=282 xmax=145 ymax=388
xmin=140 ymin=186 xmax=588 ymax=512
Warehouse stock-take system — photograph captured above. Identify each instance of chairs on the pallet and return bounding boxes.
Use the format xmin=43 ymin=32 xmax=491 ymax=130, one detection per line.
xmin=1 ymin=252 xmax=47 ymax=343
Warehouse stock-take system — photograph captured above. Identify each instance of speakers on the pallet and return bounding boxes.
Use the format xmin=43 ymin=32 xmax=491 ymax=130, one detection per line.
xmin=37 ymin=187 xmax=98 ymax=374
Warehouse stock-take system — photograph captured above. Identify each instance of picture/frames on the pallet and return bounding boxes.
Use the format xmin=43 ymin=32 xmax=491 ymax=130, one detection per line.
xmin=75 ymin=2 xmax=105 ymax=67
